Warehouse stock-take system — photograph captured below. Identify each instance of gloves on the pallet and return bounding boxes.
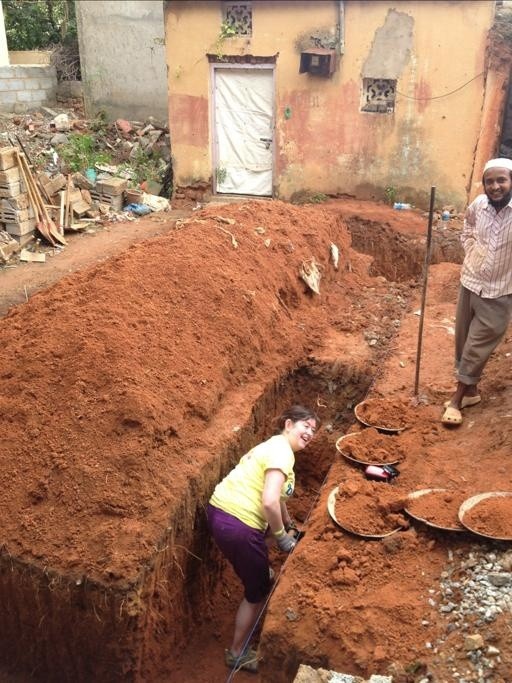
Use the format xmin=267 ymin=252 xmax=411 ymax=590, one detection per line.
xmin=277 ymin=534 xmax=298 ymax=552
xmin=284 ymin=520 xmax=301 ymax=538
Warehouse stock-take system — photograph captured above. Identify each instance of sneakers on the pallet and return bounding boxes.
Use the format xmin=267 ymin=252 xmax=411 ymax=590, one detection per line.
xmin=224 ymin=648 xmax=258 ymax=673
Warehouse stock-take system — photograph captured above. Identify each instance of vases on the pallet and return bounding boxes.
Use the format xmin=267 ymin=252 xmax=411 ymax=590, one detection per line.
xmin=126 ymin=188 xmax=141 ymax=204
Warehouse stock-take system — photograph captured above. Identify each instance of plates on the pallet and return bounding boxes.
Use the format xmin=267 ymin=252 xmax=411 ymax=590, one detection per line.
xmin=335 ymin=432 xmax=405 ymax=466
xmin=404 ymin=488 xmax=471 ymax=533
xmin=353 ymin=398 xmax=414 ymax=432
xmin=328 ymin=480 xmax=409 ymax=538
xmin=457 ymin=491 xmax=512 ymax=542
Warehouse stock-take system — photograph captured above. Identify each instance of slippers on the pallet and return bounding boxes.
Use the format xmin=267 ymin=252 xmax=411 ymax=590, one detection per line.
xmin=440 ymin=407 xmax=463 ymax=425
xmin=443 ymin=395 xmax=482 ymax=410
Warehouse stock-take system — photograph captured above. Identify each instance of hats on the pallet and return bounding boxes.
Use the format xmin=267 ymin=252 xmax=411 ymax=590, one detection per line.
xmin=482 ymin=157 xmax=512 ymax=177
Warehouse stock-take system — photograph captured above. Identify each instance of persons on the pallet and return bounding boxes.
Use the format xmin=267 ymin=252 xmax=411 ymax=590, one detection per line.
xmin=436 ymin=155 xmax=512 ymax=427
xmin=207 ymin=403 xmax=320 ymax=675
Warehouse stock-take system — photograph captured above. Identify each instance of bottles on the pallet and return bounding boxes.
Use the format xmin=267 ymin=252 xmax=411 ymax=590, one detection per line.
xmin=393 ymin=202 xmax=412 ymax=210
xmin=441 ymin=208 xmax=450 ymax=229
